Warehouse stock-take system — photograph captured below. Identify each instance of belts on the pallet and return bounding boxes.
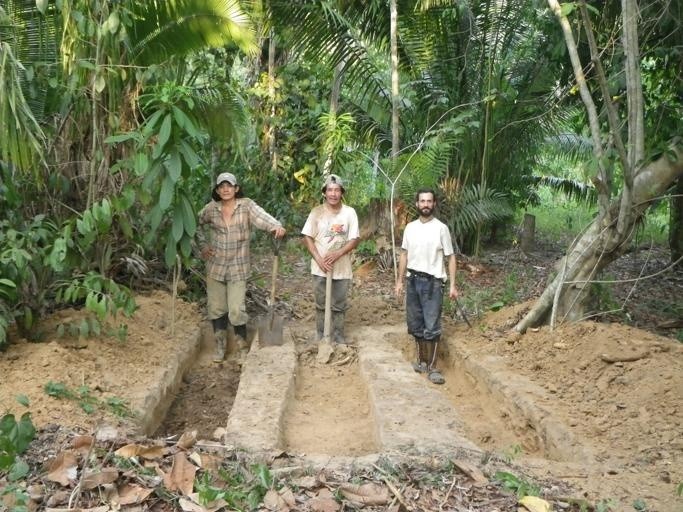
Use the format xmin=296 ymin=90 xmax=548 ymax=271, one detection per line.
xmin=413 ymin=272 xmax=435 ymax=300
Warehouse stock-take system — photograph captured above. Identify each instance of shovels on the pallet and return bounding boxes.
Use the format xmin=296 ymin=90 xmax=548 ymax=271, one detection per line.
xmin=315 ymin=251 xmax=333 ymax=364
xmin=256 ymin=231 xmax=285 ymax=347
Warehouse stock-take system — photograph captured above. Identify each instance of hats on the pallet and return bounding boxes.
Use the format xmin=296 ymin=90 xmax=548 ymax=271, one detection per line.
xmin=216 ymin=172 xmax=237 ymax=186
xmin=324 ymin=173 xmax=346 ymax=192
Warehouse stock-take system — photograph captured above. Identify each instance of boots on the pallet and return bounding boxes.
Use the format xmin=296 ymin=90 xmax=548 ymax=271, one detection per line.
xmin=210 ymin=312 xmax=228 ymax=364
xmin=309 ymin=308 xmax=325 ymax=351
xmin=425 ymin=338 xmax=445 ymax=385
xmin=331 ymin=311 xmax=348 ymax=353
xmin=231 ymin=323 xmax=248 ymax=365
xmin=411 ymin=336 xmax=429 ymax=373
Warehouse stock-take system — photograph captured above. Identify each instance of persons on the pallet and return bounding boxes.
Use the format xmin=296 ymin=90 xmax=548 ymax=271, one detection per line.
xmin=300 ymin=174 xmax=360 ymax=346
xmin=196 ymin=172 xmax=287 ymax=363
xmin=394 ymin=188 xmax=457 ymax=383
